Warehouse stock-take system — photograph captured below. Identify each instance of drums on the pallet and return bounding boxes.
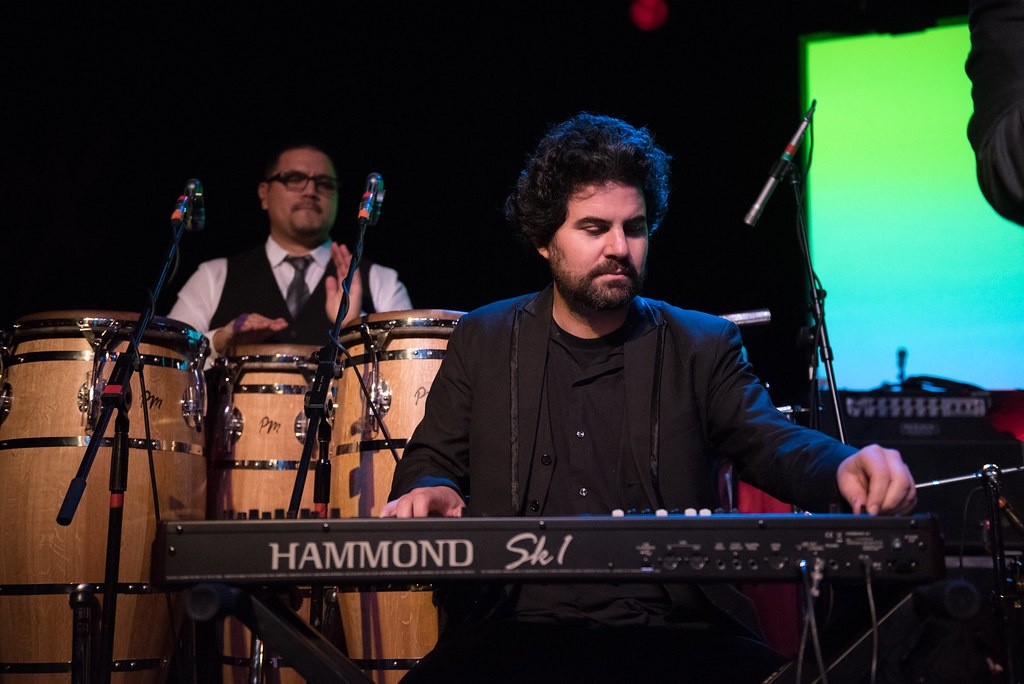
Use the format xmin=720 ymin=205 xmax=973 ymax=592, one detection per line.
xmin=0 ymin=309 xmax=214 ymax=683
xmin=327 ymin=309 xmax=465 ymax=683
xmin=214 ymin=340 xmax=342 ymax=684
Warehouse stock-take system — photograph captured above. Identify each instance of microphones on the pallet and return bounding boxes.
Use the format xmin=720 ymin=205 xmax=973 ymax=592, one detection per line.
xmin=740 ymin=105 xmax=815 ymax=228
xmin=896 ymin=345 xmax=906 ymax=371
xmin=358 ymin=170 xmax=386 ymax=227
xmin=173 ymin=175 xmax=206 ymax=233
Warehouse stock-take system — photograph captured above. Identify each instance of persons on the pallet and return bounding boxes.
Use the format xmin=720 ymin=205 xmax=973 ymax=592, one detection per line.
xmin=163 ymin=145 xmax=412 ymax=470
xmin=379 ymin=112 xmax=915 ymax=683
xmin=965 ymin=2 xmax=1024 ymax=228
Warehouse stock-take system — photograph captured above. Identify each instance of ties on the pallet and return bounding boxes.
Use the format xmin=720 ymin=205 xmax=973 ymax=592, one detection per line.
xmin=283 ymin=255 xmax=315 ymax=322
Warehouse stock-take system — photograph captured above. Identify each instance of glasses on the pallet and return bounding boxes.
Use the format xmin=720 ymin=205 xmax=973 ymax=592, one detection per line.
xmin=266 ymin=170 xmax=343 ymax=194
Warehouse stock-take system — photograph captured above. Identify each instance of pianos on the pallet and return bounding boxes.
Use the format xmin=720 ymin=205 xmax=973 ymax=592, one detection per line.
xmin=153 ymin=506 xmax=953 ymax=594
